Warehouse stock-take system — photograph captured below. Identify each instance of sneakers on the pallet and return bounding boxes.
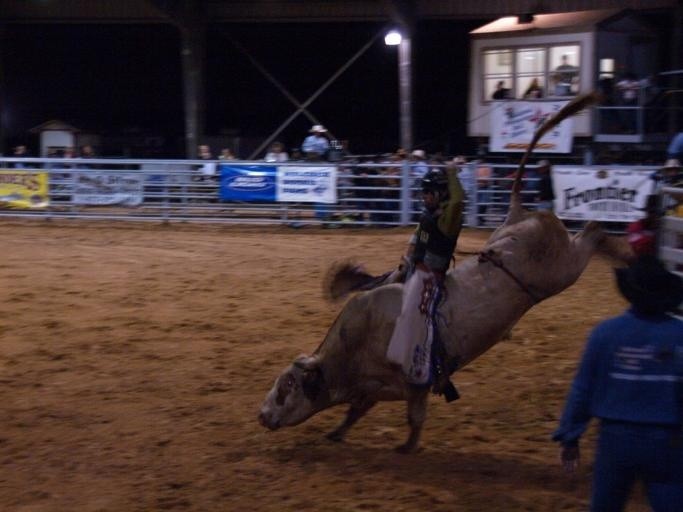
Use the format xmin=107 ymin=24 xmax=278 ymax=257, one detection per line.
xmin=431 ymin=353 xmax=458 ymax=394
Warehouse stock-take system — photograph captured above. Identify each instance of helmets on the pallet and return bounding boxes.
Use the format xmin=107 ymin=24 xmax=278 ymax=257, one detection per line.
xmin=422 ymin=168 xmax=449 ymax=185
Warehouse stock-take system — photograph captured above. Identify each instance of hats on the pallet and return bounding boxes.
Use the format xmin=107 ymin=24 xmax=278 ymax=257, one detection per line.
xmin=614 ymin=256 xmax=682 ymax=310
xmin=635 ymin=195 xmax=659 ymax=213
xmin=413 ymin=150 xmax=427 ymax=159
xmin=658 ymin=159 xmax=680 ymax=170
xmin=309 ymin=125 xmax=327 ymax=133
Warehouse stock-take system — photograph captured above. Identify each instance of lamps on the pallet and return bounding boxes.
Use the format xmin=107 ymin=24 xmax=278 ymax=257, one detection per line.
xmin=382 ymin=21 xmax=401 ymax=46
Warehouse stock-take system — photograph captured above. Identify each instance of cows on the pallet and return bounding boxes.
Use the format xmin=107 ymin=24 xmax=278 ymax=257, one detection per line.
xmin=259 ymin=85 xmax=636 ymax=454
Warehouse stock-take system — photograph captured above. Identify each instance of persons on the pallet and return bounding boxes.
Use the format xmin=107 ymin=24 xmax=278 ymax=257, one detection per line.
xmin=492 ymin=81 xmax=510 ymax=99
xmin=401 ymin=160 xmax=465 ymax=403
xmin=551 ymin=254 xmax=682 ymax=512
xmin=616 ymin=72 xmax=661 ymax=135
xmin=524 ymin=79 xmax=541 ymax=99
xmin=11 ymin=143 xmax=98 ymax=220
xmin=612 ymin=73 xmax=637 ymax=134
xmin=197 ymin=125 xmax=682 ymax=239
